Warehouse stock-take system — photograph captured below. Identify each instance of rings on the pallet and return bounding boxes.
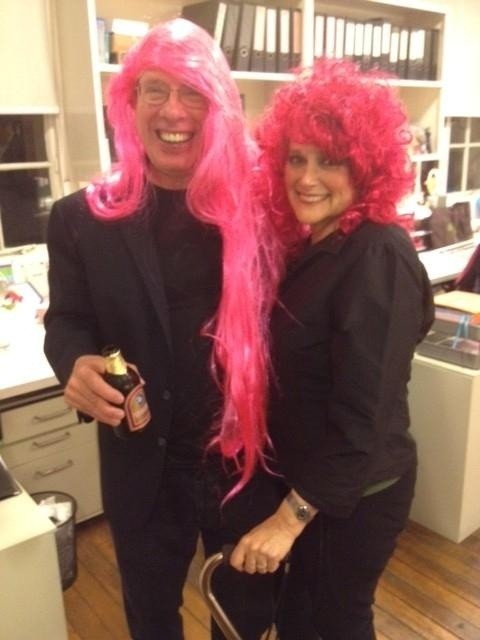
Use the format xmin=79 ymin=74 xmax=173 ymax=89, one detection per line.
xmin=257 ymin=565 xmax=268 ymax=569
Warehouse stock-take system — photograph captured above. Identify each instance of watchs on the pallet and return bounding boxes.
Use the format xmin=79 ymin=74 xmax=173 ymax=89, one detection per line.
xmin=288 ymin=493 xmax=312 ymax=520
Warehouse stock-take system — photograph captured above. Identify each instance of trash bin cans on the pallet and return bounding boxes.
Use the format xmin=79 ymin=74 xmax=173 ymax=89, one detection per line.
xmin=29 ymin=491 xmax=78 ymax=592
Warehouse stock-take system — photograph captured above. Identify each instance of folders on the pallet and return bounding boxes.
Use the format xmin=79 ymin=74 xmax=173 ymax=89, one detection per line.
xmin=183 ymin=0 xmax=444 ymax=79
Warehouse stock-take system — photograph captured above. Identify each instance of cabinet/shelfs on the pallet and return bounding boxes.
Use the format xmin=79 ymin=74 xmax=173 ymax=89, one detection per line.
xmin=51 ymin=0 xmax=450 ymax=213
xmin=0 ymin=376 xmax=106 ymax=640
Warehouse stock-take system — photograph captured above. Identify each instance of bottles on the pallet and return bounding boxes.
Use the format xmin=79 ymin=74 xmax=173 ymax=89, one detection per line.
xmin=99 ymin=343 xmax=158 ymax=457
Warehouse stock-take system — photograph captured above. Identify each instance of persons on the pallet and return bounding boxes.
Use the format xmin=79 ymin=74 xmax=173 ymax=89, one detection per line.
xmin=217 ymin=59 xmax=436 ymax=640
xmin=43 ymin=19 xmax=288 ymax=640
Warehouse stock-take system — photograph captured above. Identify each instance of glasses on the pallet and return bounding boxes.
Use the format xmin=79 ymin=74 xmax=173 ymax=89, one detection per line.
xmin=135 ymin=79 xmax=206 ymax=109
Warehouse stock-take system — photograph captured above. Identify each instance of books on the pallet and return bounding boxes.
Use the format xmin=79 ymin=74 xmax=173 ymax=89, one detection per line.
xmin=417 ymin=289 xmax=480 ymax=369
xmin=183 ymin=0 xmax=440 ymax=80
